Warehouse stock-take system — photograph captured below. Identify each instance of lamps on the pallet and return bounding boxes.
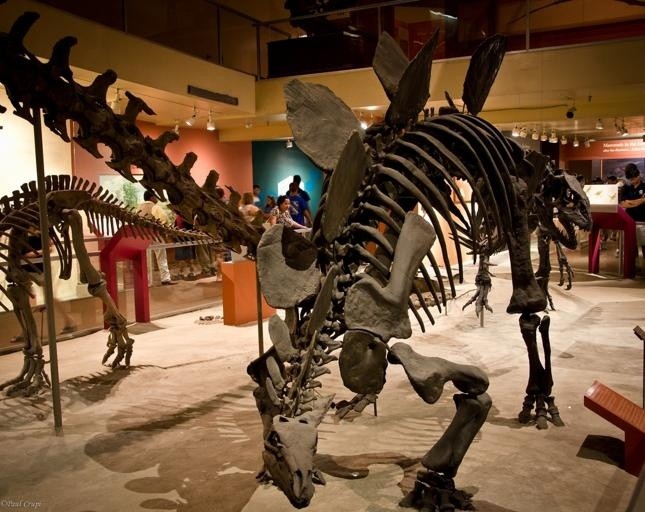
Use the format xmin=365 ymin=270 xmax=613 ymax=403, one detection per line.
xmin=206 ymin=106 xmax=215 ymax=130
xmin=595 ymin=116 xmax=604 ymax=129
xmin=512 ymin=123 xmax=579 ymax=147
xmin=185 ymin=103 xmax=197 ymax=127
xmin=110 ymin=87 xmax=120 ymax=114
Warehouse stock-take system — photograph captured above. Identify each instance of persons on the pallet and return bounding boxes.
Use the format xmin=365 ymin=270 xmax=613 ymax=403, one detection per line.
xmin=592 ymin=163 xmax=645 ymax=259
xmin=136 ymin=175 xmax=313 ymax=285
